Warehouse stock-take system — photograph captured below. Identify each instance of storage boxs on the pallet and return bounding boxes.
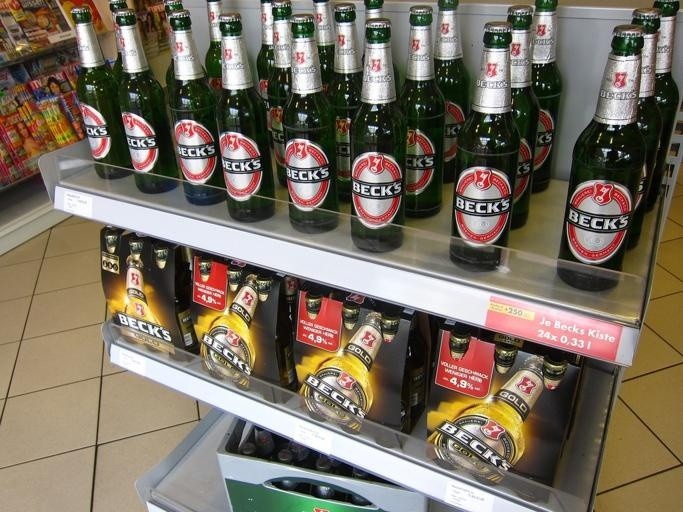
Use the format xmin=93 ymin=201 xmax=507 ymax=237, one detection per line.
xmin=192 ymin=255 xmax=298 ymax=404
xmin=216 ymin=418 xmax=428 ymax=512
xmin=99 ymin=226 xmax=198 ymax=362
xmin=294 ymin=282 xmax=427 ymax=450
xmin=426 ymin=319 xmax=585 ymax=503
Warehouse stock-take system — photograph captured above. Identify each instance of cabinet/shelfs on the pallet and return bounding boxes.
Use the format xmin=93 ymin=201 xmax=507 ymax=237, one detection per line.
xmin=39 ymin=0 xmax=683 ymax=512
xmin=0 ymin=39 xmax=86 ymax=191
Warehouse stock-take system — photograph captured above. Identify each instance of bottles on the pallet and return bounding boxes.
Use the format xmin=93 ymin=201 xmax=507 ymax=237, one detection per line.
xmin=107 ymin=0 xmax=155 ymax=85
xmin=199 ymin=273 xmax=258 ymax=392
xmin=69 ymin=5 xmax=134 ymax=180
xmin=166 ymin=0 xmax=274 ymax=206
xmin=115 ymin=9 xmax=180 ymax=194
xmin=122 ymin=255 xmax=167 ymax=354
xmin=426 ymin=354 xmax=545 ymax=487
xmin=241 ymin=426 xmax=372 ymax=508
xmin=104 ymin=230 xmax=200 ymax=360
xmin=450 ymin=318 xmax=584 ymax=500
xmin=296 ymin=311 xmax=382 ymax=434
xmin=199 ymin=257 xmax=299 ymax=393
xmin=304 ymin=282 xmax=428 ymax=446
xmin=349 ymin=0 xmax=470 ymax=253
xmin=281 ymin=0 xmax=395 ymax=234
xmin=215 ymin=0 xmax=334 ymax=222
xmin=558 ymin=0 xmax=683 ymax=291
xmin=450 ymin=0 xmax=561 ymax=273
